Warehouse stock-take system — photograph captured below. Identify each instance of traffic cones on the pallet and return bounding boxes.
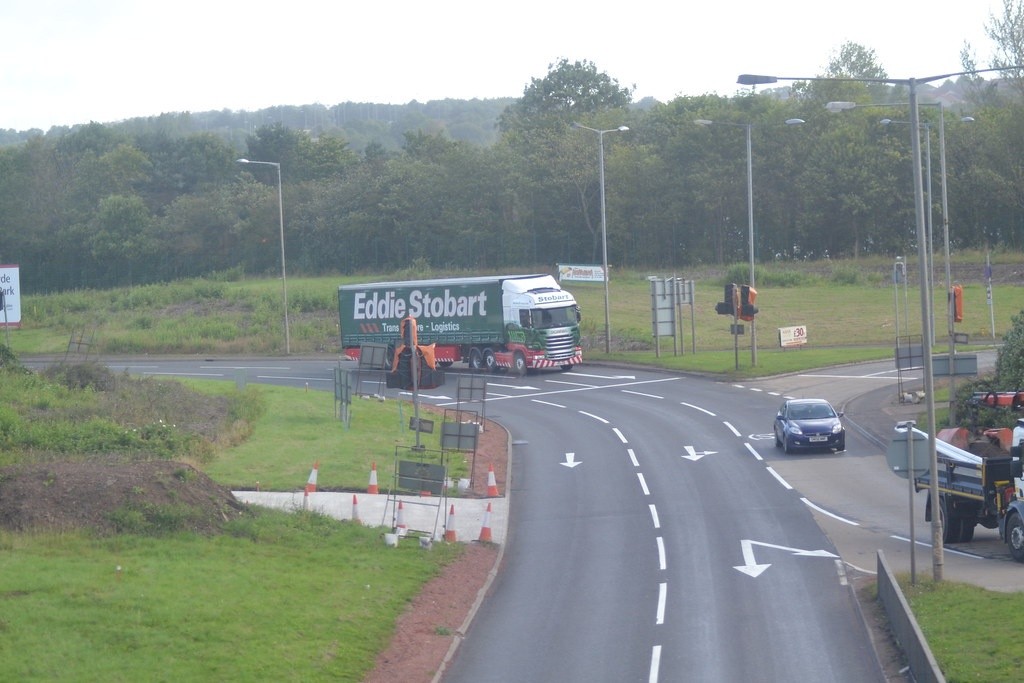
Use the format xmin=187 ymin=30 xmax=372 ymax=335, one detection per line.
xmin=481 ymin=463 xmax=504 ymax=499
xmin=395 ymin=501 xmax=406 ymax=529
xmin=305 ymin=461 xmax=318 ymax=492
xmin=303 ymin=486 xmax=311 ymax=510
xmin=366 ymin=462 xmax=379 ymax=494
xmin=471 ymin=502 xmax=496 ymax=545
xmin=443 ymin=503 xmax=457 ymax=542
xmin=418 ymin=490 xmax=433 ymax=497
xmin=351 ymin=494 xmax=362 ymax=525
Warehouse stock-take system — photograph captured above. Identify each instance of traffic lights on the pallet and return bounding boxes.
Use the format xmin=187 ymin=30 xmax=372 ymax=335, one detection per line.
xmin=715 ymin=283 xmax=736 ymax=316
xmin=738 ymin=284 xmax=759 ymax=322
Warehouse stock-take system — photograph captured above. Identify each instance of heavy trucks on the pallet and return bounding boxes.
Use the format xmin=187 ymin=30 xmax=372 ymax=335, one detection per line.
xmin=338 ymin=274 xmax=582 ymax=377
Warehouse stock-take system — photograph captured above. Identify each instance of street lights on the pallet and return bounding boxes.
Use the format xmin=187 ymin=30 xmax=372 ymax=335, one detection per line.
xmin=880 ymin=115 xmax=975 ymax=347
xmin=567 ymin=122 xmax=629 ymax=354
xmin=236 ymin=158 xmax=290 ymax=358
xmin=822 ymin=100 xmax=957 ymax=379
xmin=736 ymin=66 xmax=1023 ymax=583
xmin=693 ymin=118 xmax=806 ymax=370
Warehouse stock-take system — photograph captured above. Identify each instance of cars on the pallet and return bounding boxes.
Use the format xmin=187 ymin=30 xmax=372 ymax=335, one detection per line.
xmin=775 ymin=398 xmax=846 ymax=453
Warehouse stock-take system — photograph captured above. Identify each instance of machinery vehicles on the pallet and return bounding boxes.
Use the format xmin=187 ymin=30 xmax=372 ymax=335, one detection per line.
xmin=914 ymin=389 xmax=1024 ymax=563
